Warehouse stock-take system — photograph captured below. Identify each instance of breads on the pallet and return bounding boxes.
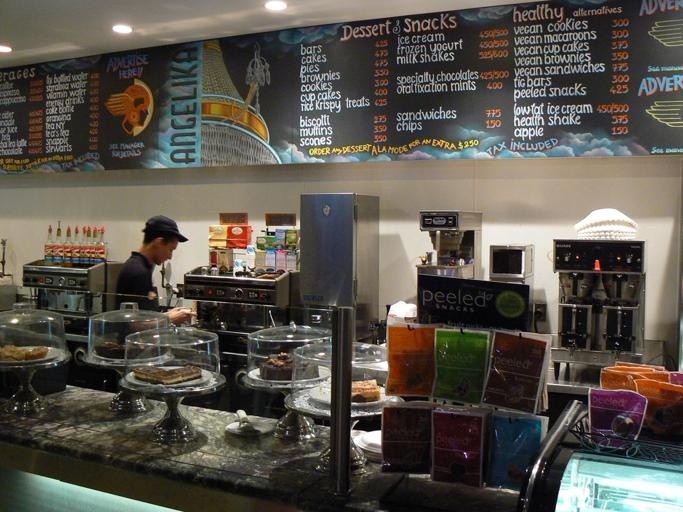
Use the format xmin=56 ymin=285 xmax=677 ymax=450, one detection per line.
xmin=259 ymin=354 xmax=320 ymax=380
xmin=350 ymin=377 xmax=382 ymax=400
xmin=132 ymin=365 xmax=202 ymax=384
xmin=94 ymin=342 xmax=166 ymax=359
xmin=0 ymin=345 xmax=49 ymax=360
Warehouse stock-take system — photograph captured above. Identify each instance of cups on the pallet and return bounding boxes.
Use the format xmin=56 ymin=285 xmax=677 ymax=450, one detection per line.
xmin=70 ymin=295 xmax=81 ymax=312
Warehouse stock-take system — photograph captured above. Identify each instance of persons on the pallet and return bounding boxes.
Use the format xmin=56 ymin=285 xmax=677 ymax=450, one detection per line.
xmin=113 ymin=215 xmax=198 ymax=346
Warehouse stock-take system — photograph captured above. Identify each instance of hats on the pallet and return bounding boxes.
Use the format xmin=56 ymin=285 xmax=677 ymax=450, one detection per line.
xmin=143 ymin=216 xmax=187 ymax=242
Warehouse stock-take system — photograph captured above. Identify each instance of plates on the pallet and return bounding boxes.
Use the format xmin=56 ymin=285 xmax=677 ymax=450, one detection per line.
xmin=308 ymin=385 xmax=392 ymax=408
xmin=91 ymin=341 xmax=170 ymax=362
xmin=124 ymin=365 xmax=212 ymax=388
xmin=248 ymin=363 xmax=332 ymax=385
xmin=0 ymin=345 xmax=58 ymax=362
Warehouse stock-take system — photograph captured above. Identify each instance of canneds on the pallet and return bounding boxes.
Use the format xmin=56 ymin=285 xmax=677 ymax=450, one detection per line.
xmin=201 ymin=266 xmax=210 ymax=275
xmin=210 ymin=267 xmax=219 ymax=276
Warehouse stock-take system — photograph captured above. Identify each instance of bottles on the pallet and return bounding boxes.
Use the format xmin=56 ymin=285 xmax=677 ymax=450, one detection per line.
xmin=43 ymin=225 xmax=107 ymax=265
